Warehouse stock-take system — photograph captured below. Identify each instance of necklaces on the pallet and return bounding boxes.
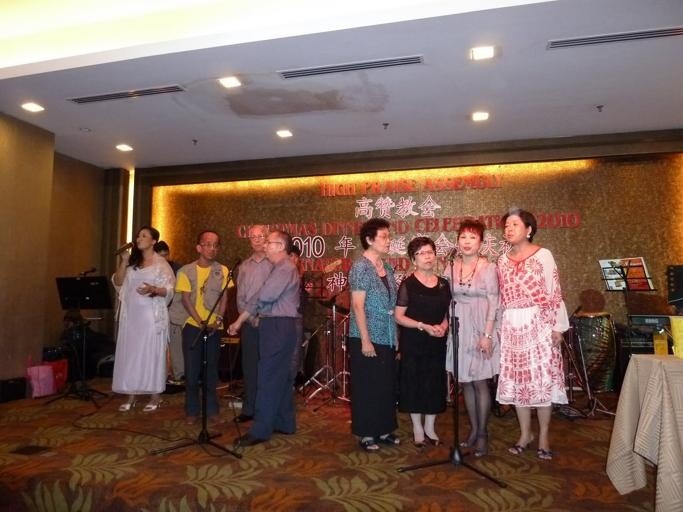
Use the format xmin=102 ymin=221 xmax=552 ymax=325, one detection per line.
xmin=460 ymin=256 xmax=479 ymax=288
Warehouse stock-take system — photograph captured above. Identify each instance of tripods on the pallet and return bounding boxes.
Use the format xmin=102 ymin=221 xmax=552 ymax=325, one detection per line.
xmin=298 ymin=338 xmax=352 ymax=412
xmin=575 ymin=367 xmax=614 ymax=419
xmin=146 ymin=359 xmax=245 ymax=464
xmin=396 ymin=330 xmax=508 ymax=493
xmin=42 ymin=333 xmax=108 ymax=409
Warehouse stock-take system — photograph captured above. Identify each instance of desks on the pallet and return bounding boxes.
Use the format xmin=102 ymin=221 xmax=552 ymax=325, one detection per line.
xmin=604 ymin=350 xmax=683 ymax=512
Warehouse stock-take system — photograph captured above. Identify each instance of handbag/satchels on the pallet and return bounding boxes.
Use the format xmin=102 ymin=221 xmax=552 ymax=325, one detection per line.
xmin=23 ymin=354 xmax=69 ymax=399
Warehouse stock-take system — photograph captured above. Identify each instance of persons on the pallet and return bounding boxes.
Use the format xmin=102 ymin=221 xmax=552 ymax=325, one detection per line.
xmin=347 ymin=217 xmax=399 ymax=451
xmin=439 ymin=220 xmax=500 ymax=457
xmin=496 ymin=210 xmax=569 ymax=461
xmin=173 ymin=233 xmax=234 ymax=423
xmin=226 ymin=226 xmax=305 ymax=447
xmin=394 ymin=236 xmax=452 ymax=448
xmin=111 ymin=227 xmax=185 ymax=412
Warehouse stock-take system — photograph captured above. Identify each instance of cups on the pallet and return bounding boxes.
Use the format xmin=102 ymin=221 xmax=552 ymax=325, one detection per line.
xmin=668 ymin=314 xmax=683 ymax=358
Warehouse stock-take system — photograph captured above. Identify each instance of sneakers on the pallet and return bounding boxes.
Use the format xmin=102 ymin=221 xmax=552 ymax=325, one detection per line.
xmin=184 ymin=413 xmax=200 ymax=427
xmin=207 ymin=412 xmax=229 ymax=424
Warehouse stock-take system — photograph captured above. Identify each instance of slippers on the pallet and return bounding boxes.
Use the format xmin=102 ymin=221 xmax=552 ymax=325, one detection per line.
xmin=506 ymin=437 xmax=531 ymax=455
xmin=537 ymin=447 xmax=554 ymax=462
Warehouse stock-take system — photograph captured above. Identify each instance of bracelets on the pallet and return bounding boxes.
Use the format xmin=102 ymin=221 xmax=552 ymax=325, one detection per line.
xmin=483 ymin=333 xmax=493 ymax=339
xmin=418 ymin=322 xmax=423 ymax=330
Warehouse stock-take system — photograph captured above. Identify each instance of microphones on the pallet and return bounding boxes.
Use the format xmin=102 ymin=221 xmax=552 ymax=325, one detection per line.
xmin=113 ymin=241 xmax=134 ymax=257
xmin=79 ymin=266 xmax=97 ymax=278
xmin=227 ymin=255 xmax=245 ymax=279
xmin=444 ymin=245 xmax=463 ymax=266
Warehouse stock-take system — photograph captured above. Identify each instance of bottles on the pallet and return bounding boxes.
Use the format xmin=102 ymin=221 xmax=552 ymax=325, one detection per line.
xmin=652 ymin=324 xmax=669 ymax=360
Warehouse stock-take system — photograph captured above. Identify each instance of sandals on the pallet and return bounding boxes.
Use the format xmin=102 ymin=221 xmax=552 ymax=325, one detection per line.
xmin=118 ymin=400 xmax=136 ymax=413
xmin=142 ymin=400 xmax=165 ymax=412
xmin=379 ymin=434 xmax=404 ymax=447
xmin=358 ymin=435 xmax=379 ymax=452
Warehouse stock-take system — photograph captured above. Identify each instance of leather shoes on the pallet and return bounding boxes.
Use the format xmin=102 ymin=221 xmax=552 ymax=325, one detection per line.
xmin=457 ymin=436 xmax=474 ymax=449
xmin=472 ymin=445 xmax=490 ymax=457
xmin=233 ymin=414 xmax=253 ymax=423
xmin=234 ymin=431 xmax=268 ymax=448
xmin=413 ymin=432 xmax=426 ymax=451
xmin=424 ymin=429 xmax=445 ymax=447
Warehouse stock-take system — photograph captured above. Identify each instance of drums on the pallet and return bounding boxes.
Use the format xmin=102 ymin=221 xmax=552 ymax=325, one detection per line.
xmin=576 ymin=312 xmax=615 ymax=394
